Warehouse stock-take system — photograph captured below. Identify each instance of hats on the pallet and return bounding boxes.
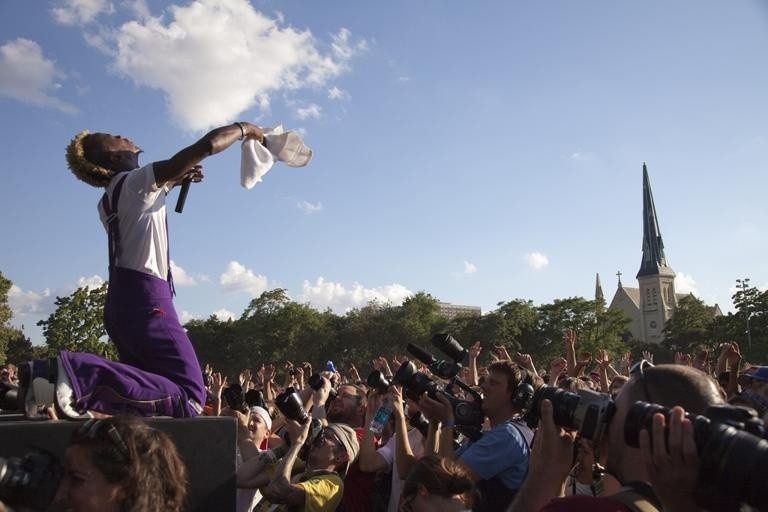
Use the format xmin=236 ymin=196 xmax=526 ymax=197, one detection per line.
xmin=249 ymin=407 xmax=273 ymax=451
xmin=737 ymin=365 xmax=768 ymax=388
xmin=325 ymin=423 xmax=361 ymax=480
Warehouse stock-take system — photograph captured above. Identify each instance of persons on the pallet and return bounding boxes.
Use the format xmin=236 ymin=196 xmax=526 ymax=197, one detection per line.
xmin=203 ymin=328 xmax=768 ymax=510
xmin=2 ymin=364 xmax=20 ymax=385
xmin=46 ymin=418 xmax=193 ymax=511
xmin=16 ymin=121 xmax=268 ymax=422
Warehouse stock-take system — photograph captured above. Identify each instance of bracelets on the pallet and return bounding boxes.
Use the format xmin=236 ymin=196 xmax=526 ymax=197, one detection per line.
xmin=234 ymin=122 xmax=245 ymax=142
xmin=163 ymin=186 xmax=170 ymax=196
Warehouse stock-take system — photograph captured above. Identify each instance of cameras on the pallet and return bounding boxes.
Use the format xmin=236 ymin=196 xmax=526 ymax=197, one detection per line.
xmin=531 ymin=383 xmax=617 ymax=446
xmin=367 ymin=370 xmax=395 ymax=393
xmin=289 ymin=370 xmax=297 ymax=376
xmin=308 ymin=374 xmax=338 ymax=400
xmin=409 ymin=411 xmax=428 ymax=438
xmin=274 ymin=387 xmax=324 ymax=448
xmin=203 ymin=372 xmax=212 ymax=386
xmin=622 ymin=400 xmax=768 ymax=511
xmin=244 ymin=389 xmax=274 ymax=419
xmin=223 ymin=382 xmax=246 ymax=414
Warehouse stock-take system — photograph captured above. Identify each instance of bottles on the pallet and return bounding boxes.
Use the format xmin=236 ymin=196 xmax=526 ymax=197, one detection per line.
xmin=369 ymin=398 xmax=396 ymax=435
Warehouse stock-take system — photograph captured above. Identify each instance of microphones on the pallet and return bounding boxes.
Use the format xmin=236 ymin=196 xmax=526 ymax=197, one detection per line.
xmin=0 ymin=444 xmax=67 ymax=512
xmin=175 ymin=167 xmax=195 ymax=214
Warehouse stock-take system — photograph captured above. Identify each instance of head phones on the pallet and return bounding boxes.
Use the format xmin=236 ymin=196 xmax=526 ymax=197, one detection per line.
xmin=511 ymin=366 xmax=536 ymax=410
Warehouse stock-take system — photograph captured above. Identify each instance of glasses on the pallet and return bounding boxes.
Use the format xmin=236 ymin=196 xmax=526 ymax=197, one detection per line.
xmin=78 ymin=416 xmax=133 ymax=462
xmin=628 ymin=359 xmax=654 ymax=402
xmin=400 ymin=491 xmax=418 ymax=512
xmin=323 ymin=430 xmax=344 ymax=449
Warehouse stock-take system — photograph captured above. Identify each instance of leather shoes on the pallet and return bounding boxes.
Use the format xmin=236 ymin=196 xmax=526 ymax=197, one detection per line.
xmin=18 ymin=362 xmax=50 ymax=420
xmin=47 ymin=355 xmax=95 ymax=421
